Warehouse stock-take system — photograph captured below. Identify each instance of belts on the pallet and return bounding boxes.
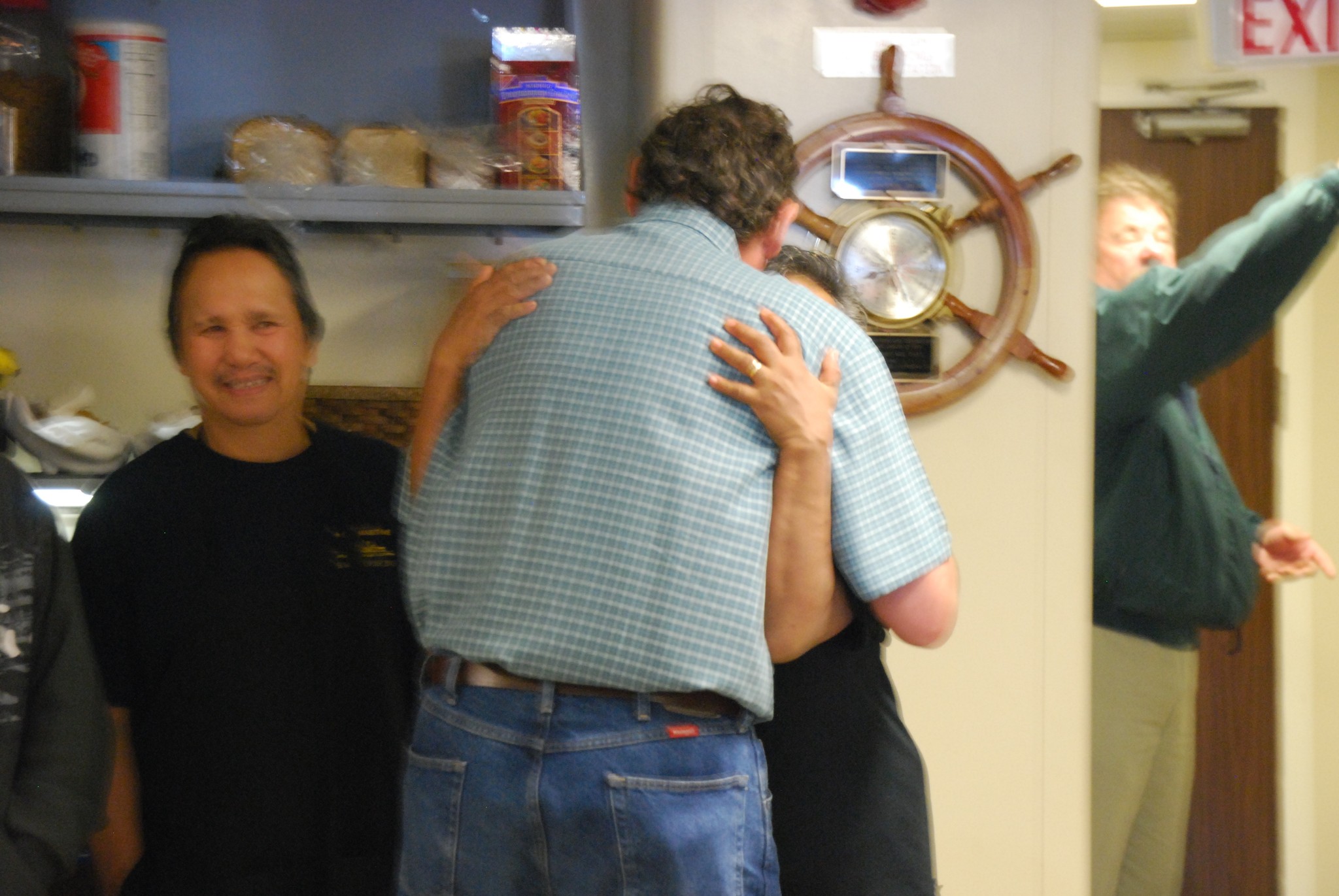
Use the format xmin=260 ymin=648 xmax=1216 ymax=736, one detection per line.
xmin=426 ymin=655 xmax=740 ymax=719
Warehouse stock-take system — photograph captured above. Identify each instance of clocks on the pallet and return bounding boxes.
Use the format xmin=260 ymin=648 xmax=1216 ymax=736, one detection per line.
xmin=832 ymin=205 xmax=954 ymax=335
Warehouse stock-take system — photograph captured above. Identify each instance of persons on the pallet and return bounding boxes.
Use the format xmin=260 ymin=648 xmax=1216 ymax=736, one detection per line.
xmin=74 ymin=217 xmax=408 ymax=896
xmin=411 ymin=243 xmax=935 ymax=894
xmin=0 ymin=427 xmax=110 ymax=896
xmin=390 ymin=77 xmax=960 ymax=896
xmin=1093 ymin=160 xmax=1339 ymax=896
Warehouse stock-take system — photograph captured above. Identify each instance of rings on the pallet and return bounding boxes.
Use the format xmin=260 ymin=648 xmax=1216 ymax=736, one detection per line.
xmin=746 ymin=359 xmax=759 ymax=376
